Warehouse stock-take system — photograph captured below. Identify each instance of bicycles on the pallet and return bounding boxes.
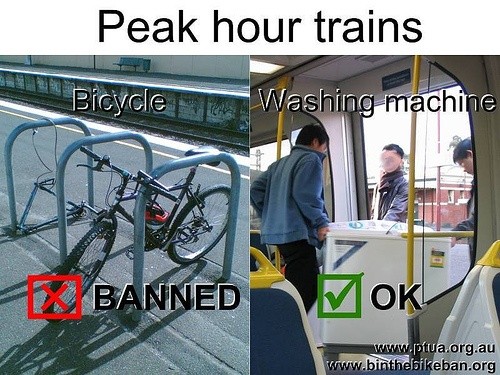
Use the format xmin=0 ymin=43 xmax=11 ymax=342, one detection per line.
xmin=42 ymin=144 xmax=234 ymax=323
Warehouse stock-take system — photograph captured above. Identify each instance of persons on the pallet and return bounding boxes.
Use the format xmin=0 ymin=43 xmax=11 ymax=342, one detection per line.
xmin=448 ymin=138 xmax=476 ymax=258
xmin=250 ymin=123 xmax=333 ymax=315
xmin=369 ymin=143 xmax=409 ymax=222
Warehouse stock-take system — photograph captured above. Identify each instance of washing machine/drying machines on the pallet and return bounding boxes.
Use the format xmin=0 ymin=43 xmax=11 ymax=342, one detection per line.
xmin=320 ymin=219 xmax=453 ymax=362
